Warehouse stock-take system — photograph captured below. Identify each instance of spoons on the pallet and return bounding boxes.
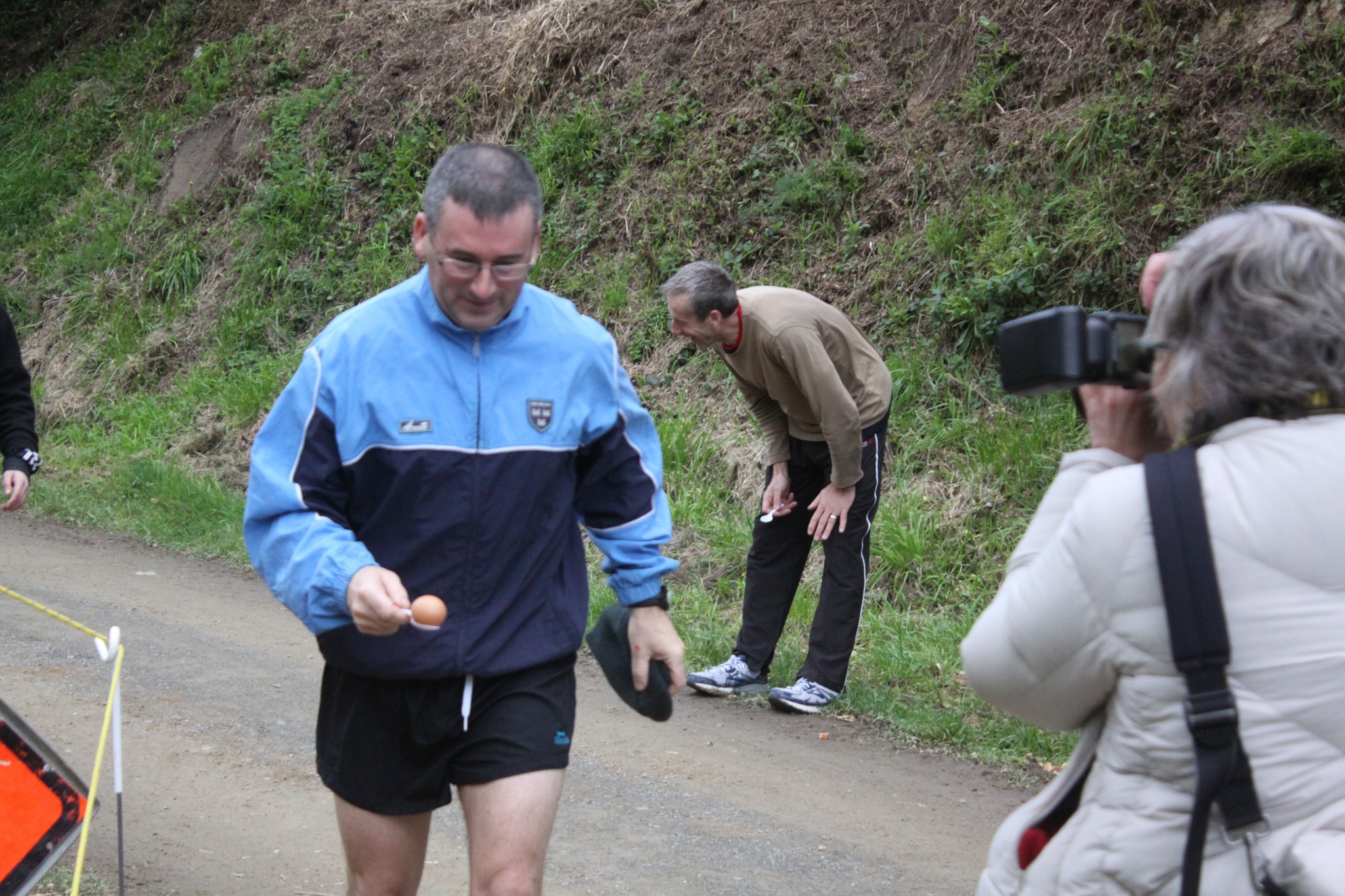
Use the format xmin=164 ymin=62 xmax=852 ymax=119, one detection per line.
xmin=402 ymin=609 xmax=441 ymax=631
xmin=760 ymin=502 xmax=785 ymax=523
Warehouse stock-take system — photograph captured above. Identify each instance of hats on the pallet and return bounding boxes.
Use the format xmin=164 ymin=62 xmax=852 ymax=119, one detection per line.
xmin=587 ymin=604 xmax=673 ymax=724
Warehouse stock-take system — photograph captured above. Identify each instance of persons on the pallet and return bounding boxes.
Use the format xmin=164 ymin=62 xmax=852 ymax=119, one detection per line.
xmin=961 ymin=204 xmax=1345 ymax=896
xmin=244 ymin=146 xmax=685 ymax=896
xmin=662 ymin=261 xmax=892 ymax=715
xmin=0 ymin=294 xmax=41 ymax=510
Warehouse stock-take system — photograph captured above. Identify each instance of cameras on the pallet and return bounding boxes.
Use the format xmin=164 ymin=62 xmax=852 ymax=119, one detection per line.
xmin=993 ymin=304 xmax=1155 ymax=423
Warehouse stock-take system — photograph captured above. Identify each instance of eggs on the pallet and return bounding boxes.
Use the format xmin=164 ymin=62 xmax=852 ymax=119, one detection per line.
xmin=411 ymin=595 xmax=447 ymax=626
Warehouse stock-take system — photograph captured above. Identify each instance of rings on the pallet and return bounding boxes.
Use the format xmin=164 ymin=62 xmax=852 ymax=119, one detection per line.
xmin=830 ymin=513 xmax=836 ymax=518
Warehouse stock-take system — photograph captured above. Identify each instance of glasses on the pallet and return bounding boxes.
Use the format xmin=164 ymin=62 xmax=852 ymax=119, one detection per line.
xmin=427 ymin=225 xmax=539 ymax=280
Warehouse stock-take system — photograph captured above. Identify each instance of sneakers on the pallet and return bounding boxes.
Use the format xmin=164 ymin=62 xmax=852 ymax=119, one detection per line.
xmin=684 ymin=653 xmax=771 ymax=695
xmin=768 ymin=678 xmax=838 ymax=714
